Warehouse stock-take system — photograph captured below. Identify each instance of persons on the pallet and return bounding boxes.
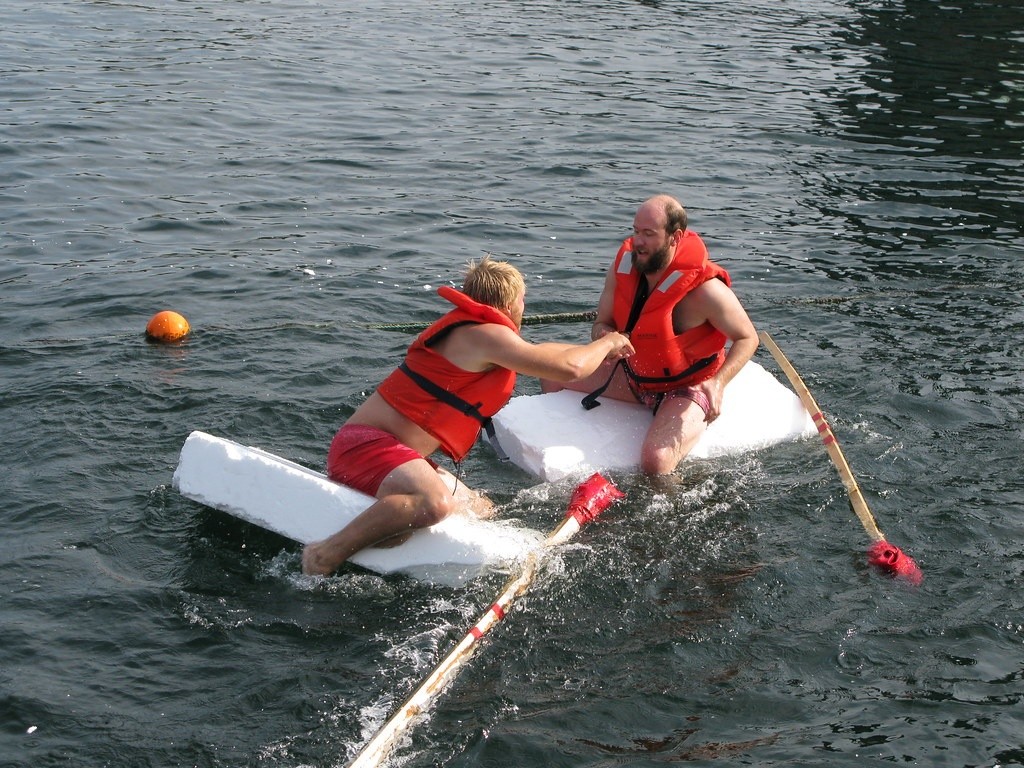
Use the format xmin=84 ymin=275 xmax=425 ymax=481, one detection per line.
xmin=535 ymin=194 xmax=759 ymax=475
xmin=301 ymin=259 xmax=636 ymax=575
